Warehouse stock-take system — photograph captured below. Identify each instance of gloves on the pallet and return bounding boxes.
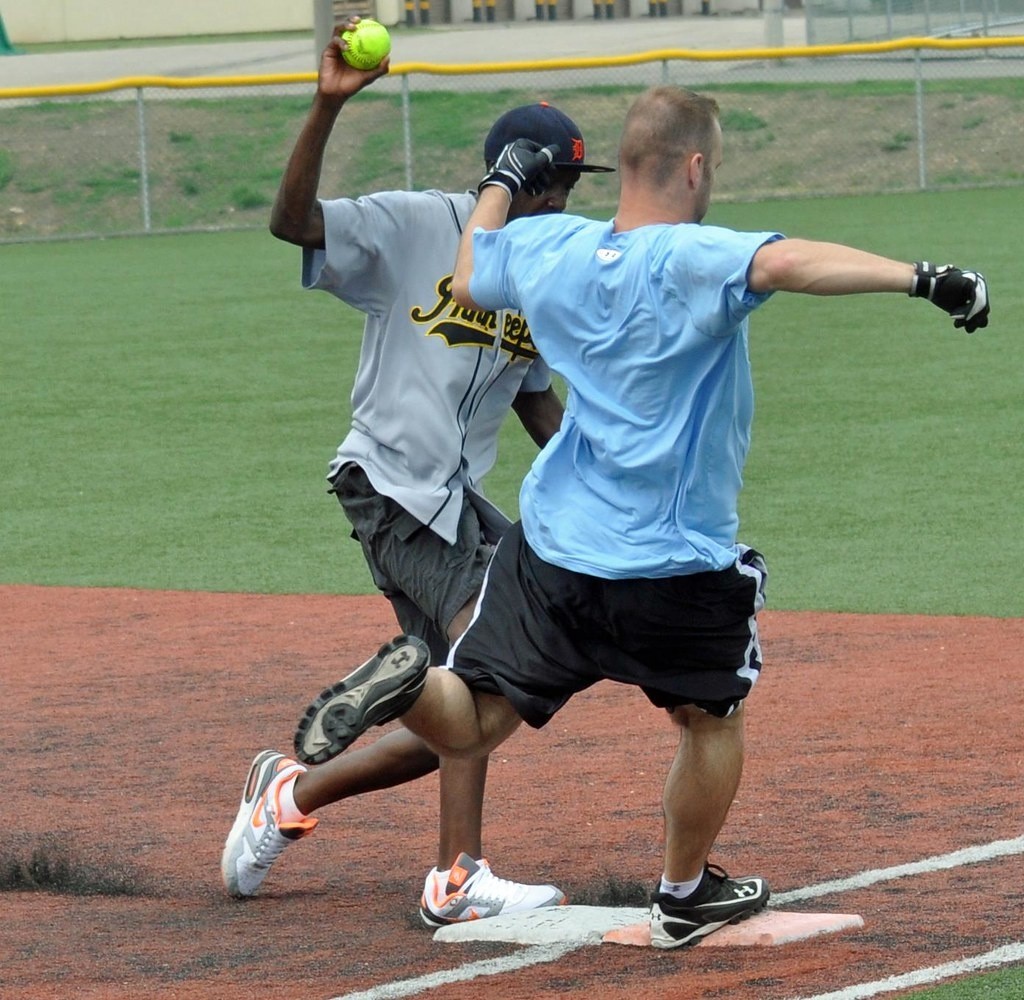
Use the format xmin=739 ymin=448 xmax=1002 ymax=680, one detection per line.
xmin=908 ymin=261 xmax=990 ymax=333
xmin=479 ymin=137 xmax=561 ymax=204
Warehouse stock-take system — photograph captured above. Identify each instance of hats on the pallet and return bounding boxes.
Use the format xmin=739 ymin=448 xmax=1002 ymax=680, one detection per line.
xmin=482 ymin=100 xmax=616 ymax=174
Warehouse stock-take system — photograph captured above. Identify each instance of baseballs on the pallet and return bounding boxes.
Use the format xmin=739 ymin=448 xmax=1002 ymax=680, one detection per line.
xmin=341 ymin=18 xmax=394 ymax=70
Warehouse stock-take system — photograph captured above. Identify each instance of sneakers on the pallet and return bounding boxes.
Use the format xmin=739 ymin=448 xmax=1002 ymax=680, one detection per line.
xmin=419 ymin=851 xmax=567 ymax=927
xmin=220 ymin=749 xmax=320 ymax=898
xmin=648 ymin=861 xmax=771 ymax=952
xmin=293 ymin=633 xmax=432 ymax=766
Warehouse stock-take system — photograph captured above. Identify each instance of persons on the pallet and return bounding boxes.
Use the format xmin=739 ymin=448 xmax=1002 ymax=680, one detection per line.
xmin=220 ymin=14 xmax=616 ymax=927
xmin=294 ymin=86 xmax=990 ymax=949
